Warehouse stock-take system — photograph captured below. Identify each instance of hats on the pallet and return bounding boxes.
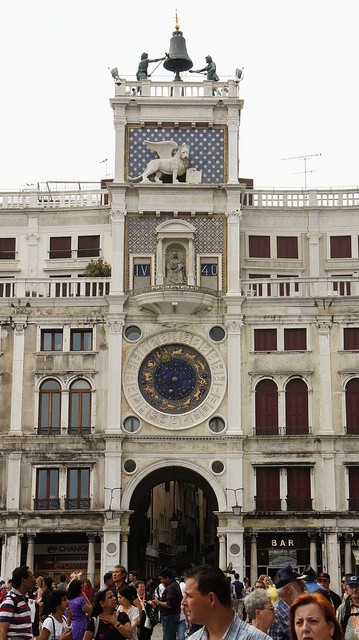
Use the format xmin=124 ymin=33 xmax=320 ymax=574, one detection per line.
xmin=273 ymin=564 xmax=308 ymax=589
xmin=302 ymin=565 xmax=319 ymax=581
xmin=346 ymin=573 xmax=358 ymax=585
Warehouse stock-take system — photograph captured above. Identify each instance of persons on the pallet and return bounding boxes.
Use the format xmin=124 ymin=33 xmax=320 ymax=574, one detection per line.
xmin=112 ymin=564 xmax=142 ymax=626
xmin=242 ymin=576 xmax=253 ymax=624
xmin=56 ymin=575 xmax=68 ymax=591
xmin=266 ymin=563 xmax=308 ymax=640
xmin=0 ymin=565 xmax=34 ymax=640
xmin=36 ymin=577 xmax=55 ymax=620
xmin=339 ymin=574 xmax=355 ymax=603
xmin=183 ymin=562 xmax=273 ymax=640
xmin=227 ymin=576 xmax=237 ymax=608
xmin=289 ymin=592 xmax=345 ymax=640
xmin=65 ymin=579 xmax=93 ymax=640
xmin=196 ymin=55 xmax=221 ymax=96
xmin=51 ymin=581 xmax=58 ymax=591
xmin=336 ymin=575 xmax=359 ymax=640
xmin=155 ymin=574 xmax=165 ymax=623
xmin=41 ymin=577 xmax=53 ymax=600
xmin=7 ymin=579 xmax=12 ymax=592
xmin=128 ymin=571 xmax=144 ymax=588
xmin=34 ymin=576 xmax=43 ymax=629
xmin=254 ymin=574 xmax=274 ymax=590
xmin=301 ymin=565 xmax=331 ymax=605
xmin=317 ymin=572 xmax=342 ymax=612
xmin=151 ymin=569 xmax=183 ymax=640
xmin=243 ymin=589 xmax=275 ymax=634
xmin=83 ymin=579 xmax=94 ymax=602
xmin=232 ymin=573 xmax=245 ymax=619
xmin=0 ymin=579 xmax=8 ymax=604
xmin=97 ymin=573 xmax=115 ymax=592
xmin=166 ymin=252 xmax=185 ymax=284
xmin=175 ymin=576 xmax=187 ymax=640
xmin=135 ymin=581 xmax=156 ymax=640
xmin=117 ymin=588 xmax=140 ymax=640
xmin=132 ymin=53 xmax=165 ymax=95
xmin=82 ymin=588 xmax=133 ymax=640
xmin=145 ymin=577 xmax=153 ymax=591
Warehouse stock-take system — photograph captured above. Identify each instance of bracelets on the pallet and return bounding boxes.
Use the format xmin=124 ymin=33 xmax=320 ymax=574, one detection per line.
xmin=114 ymin=622 xmax=123 ymax=629
xmin=157 ymin=601 xmax=161 ymax=606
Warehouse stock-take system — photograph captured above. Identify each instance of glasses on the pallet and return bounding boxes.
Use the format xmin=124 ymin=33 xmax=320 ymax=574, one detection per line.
xmin=318 ymin=578 xmax=327 ymax=582
xmin=341 ymin=580 xmax=346 ymax=583
